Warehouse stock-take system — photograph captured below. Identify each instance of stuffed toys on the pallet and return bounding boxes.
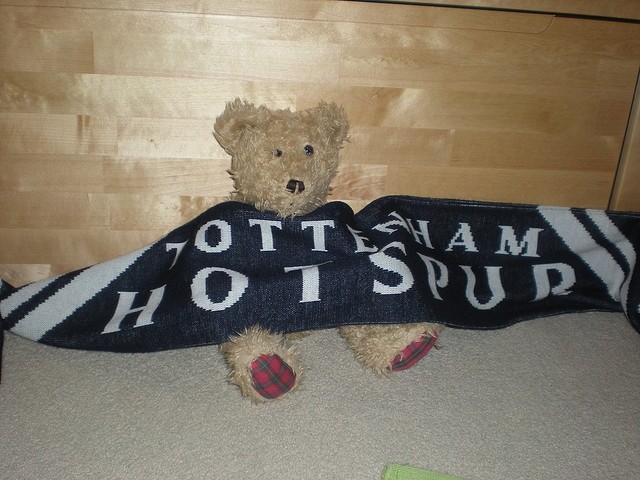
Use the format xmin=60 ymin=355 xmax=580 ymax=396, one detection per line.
xmin=212 ymin=96 xmax=438 ymax=401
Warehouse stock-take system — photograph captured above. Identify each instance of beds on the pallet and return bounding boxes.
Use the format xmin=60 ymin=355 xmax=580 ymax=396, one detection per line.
xmin=0 ymin=0 xmax=639 ymax=476
